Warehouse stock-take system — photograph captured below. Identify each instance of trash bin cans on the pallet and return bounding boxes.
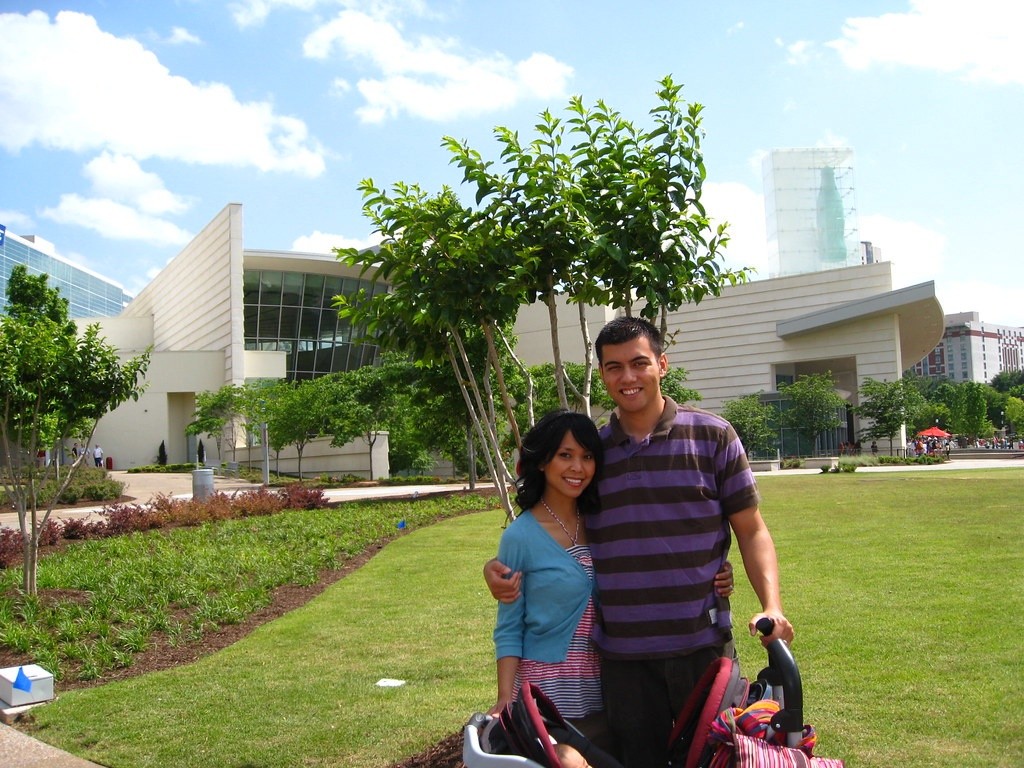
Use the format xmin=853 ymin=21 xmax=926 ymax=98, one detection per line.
xmin=227 ymin=462 xmax=239 ymax=478
xmin=192 ymin=469 xmax=214 ymax=503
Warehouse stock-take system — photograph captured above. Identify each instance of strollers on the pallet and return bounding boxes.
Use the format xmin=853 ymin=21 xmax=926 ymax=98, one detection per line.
xmin=460 ymin=617 xmax=812 ymax=768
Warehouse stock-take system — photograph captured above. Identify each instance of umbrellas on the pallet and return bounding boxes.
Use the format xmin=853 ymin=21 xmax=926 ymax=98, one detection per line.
xmin=918 ymin=427 xmax=952 ymax=437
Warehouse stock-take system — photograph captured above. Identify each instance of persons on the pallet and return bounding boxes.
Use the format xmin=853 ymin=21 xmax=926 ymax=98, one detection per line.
xmin=871 ymin=441 xmax=879 ymax=456
xmin=80 ymin=443 xmax=91 ymax=467
xmin=93 ymin=443 xmax=104 ymax=467
xmin=72 ymin=442 xmax=79 ymax=469
xmin=482 ymin=317 xmax=795 ymax=768
xmin=840 ymin=439 xmax=863 ymax=458
xmin=906 ymin=436 xmax=1024 ymax=460
